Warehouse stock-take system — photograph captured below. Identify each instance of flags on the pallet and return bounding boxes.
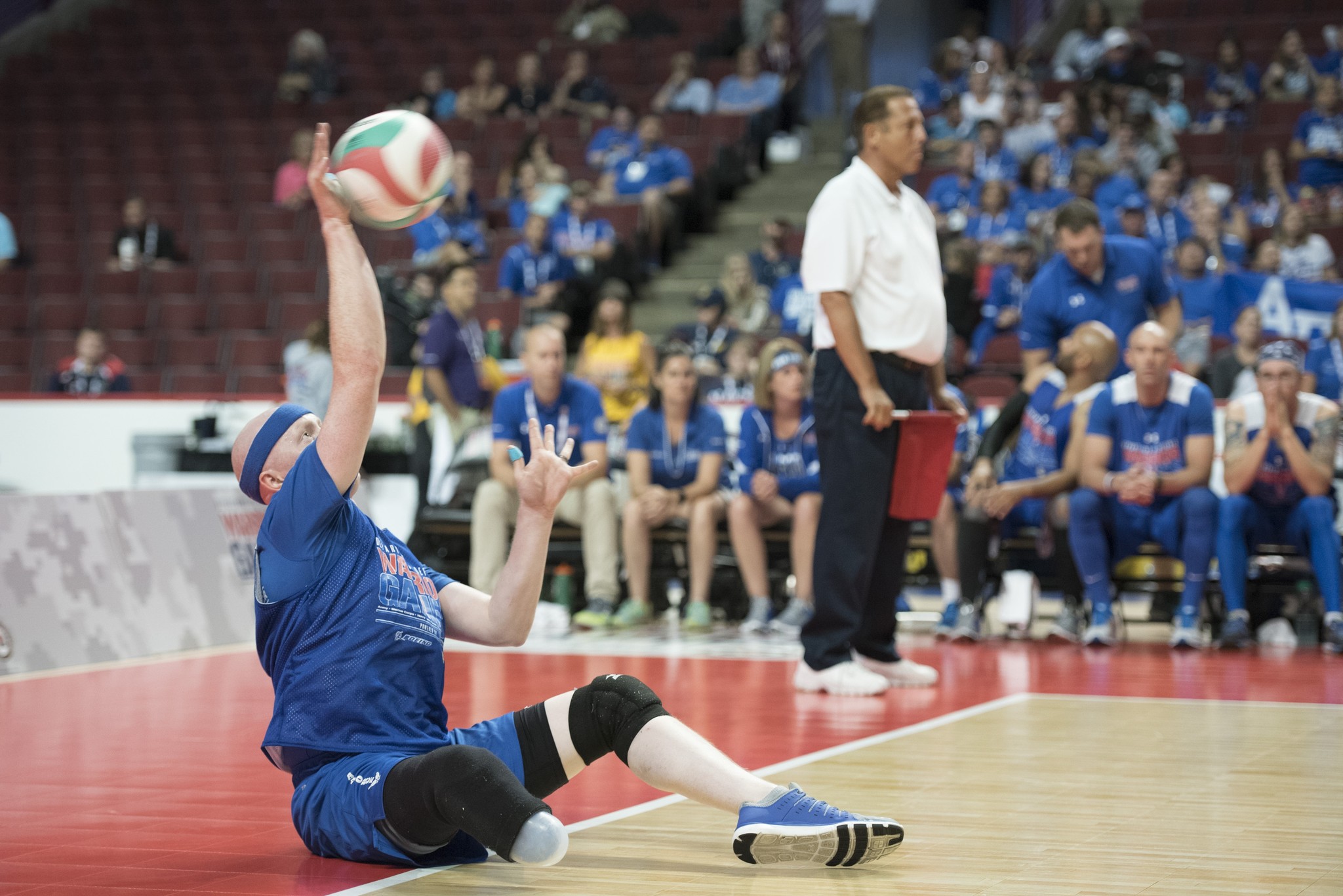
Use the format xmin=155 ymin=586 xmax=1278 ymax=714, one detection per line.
xmin=888 ymin=410 xmax=955 ymax=518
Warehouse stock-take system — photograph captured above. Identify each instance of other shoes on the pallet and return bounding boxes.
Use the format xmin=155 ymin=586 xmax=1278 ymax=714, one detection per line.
xmin=1168 ymin=619 xmax=1204 ymax=649
xmin=1220 ymin=615 xmax=1252 ymax=653
xmin=572 ymin=597 xmax=611 ymax=628
xmin=738 ymin=598 xmax=769 ymax=633
xmin=1083 ymin=608 xmax=1123 ymax=647
xmin=770 ymin=598 xmax=815 ymax=634
xmin=1045 ymin=607 xmax=1077 ymax=643
xmin=680 ymin=600 xmax=711 ymax=629
xmin=936 ymin=602 xmax=956 ymax=644
xmin=949 ymin=607 xmax=983 ymax=642
xmin=1318 ymin=616 xmax=1343 ymax=651
xmin=609 ymin=598 xmax=652 ymax=627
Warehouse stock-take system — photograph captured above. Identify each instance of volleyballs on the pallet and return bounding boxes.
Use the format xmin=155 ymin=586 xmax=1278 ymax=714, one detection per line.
xmin=330 ymin=109 xmax=457 ymax=231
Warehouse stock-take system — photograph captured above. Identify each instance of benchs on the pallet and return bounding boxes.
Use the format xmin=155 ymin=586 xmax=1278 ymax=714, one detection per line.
xmin=424 ymin=422 xmax=1343 ymax=660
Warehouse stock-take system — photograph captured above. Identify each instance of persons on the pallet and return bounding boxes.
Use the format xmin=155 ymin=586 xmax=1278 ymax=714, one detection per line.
xmin=787 ymin=85 xmax=969 ymax=694
xmin=231 ymin=123 xmax=905 ymax=867
xmin=0 ymin=1 xmax=1343 ymax=652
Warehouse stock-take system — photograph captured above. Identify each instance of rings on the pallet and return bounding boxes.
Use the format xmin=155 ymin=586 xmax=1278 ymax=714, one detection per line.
xmin=557 ymin=454 xmax=568 ymax=462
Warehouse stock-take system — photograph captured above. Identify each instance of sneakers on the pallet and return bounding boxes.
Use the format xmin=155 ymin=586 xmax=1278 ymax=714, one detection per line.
xmin=732 ymin=779 xmax=904 ymax=866
xmin=792 ymin=659 xmax=890 ymax=696
xmin=855 ymin=652 xmax=939 ymax=686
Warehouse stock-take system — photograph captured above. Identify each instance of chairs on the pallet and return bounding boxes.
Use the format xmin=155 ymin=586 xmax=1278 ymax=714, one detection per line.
xmin=0 ymin=0 xmax=1343 ymax=397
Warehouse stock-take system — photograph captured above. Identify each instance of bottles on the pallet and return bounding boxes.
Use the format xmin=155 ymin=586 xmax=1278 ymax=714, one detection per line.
xmin=549 ymin=565 xmax=574 ymax=615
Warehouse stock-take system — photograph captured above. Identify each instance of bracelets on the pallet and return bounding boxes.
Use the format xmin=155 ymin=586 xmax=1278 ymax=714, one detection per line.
xmin=1153 ymin=470 xmax=1163 ymax=495
xmin=1102 ymin=471 xmax=1114 ymax=494
xmin=676 ymin=487 xmax=685 ymax=503
